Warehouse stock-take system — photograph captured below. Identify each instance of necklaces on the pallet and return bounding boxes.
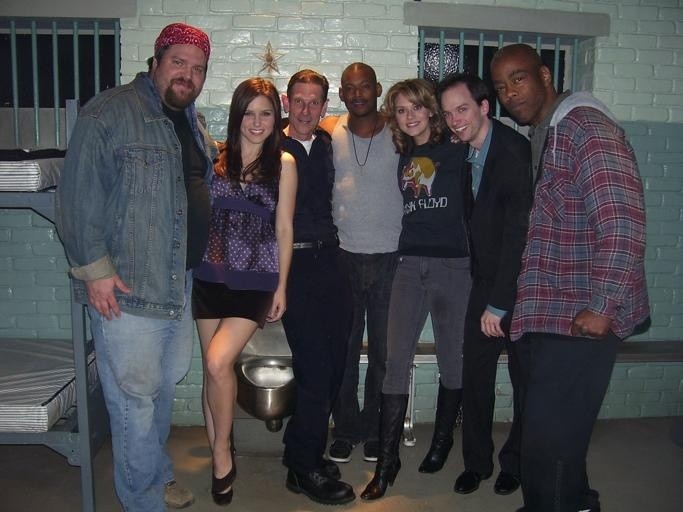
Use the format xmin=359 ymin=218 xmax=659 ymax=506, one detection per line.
xmin=348 ymin=113 xmax=380 ymax=167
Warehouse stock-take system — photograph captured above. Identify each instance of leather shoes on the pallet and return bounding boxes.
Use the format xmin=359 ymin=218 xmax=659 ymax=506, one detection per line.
xmin=212 ymin=424 xmax=236 ymax=506
xmin=283 ymin=378 xmax=521 ymax=505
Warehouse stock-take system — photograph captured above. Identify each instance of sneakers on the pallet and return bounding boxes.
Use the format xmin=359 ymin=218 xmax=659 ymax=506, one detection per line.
xmin=162 ymin=480 xmax=195 ymax=508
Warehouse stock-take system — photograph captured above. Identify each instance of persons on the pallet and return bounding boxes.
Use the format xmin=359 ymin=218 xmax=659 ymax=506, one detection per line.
xmin=437 ymin=74 xmax=534 ymax=496
xmin=192 ymin=75 xmax=299 ymax=508
xmin=357 ymin=70 xmax=477 ymax=503
xmin=50 ymin=19 xmax=219 ymax=511
xmin=212 ymin=58 xmax=405 ymax=469
xmin=274 ymin=67 xmax=359 ymax=508
xmin=488 ymin=38 xmax=655 ymax=511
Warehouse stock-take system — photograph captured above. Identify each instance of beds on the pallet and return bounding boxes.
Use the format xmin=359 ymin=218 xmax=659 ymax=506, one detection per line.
xmin=0 ymin=97 xmax=105 ymax=511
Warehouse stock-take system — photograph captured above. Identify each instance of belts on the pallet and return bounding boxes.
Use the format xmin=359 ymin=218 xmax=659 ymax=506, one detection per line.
xmin=292 ymin=235 xmax=339 ymax=252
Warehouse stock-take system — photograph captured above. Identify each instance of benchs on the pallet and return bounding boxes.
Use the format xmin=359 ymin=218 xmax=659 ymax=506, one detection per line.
xmin=358 ymin=339 xmax=682 ymax=448
